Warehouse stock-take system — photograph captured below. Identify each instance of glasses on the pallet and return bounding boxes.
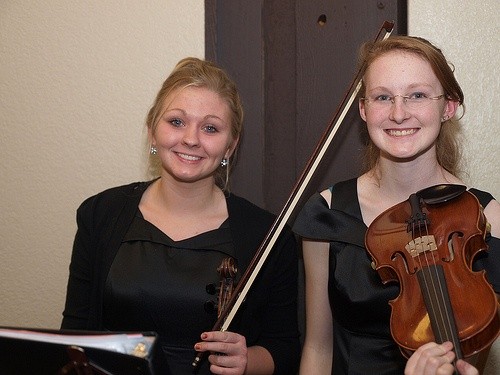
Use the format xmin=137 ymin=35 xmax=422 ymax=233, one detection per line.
xmin=359 ymin=88 xmax=446 ymax=110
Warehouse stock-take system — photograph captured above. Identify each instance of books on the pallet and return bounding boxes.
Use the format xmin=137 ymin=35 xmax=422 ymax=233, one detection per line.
xmin=0 ymin=326 xmax=169 ymax=375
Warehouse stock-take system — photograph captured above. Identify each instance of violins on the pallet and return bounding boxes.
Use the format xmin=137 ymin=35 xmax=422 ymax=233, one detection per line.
xmin=204 ymin=257 xmax=246 ymax=375
xmin=365 ymin=183 xmax=500 ymax=375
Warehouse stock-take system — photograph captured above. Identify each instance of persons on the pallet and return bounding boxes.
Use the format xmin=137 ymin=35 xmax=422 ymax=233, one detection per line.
xmin=59 ymin=57 xmax=298 ymax=375
xmin=292 ymin=36 xmax=500 ymax=375
